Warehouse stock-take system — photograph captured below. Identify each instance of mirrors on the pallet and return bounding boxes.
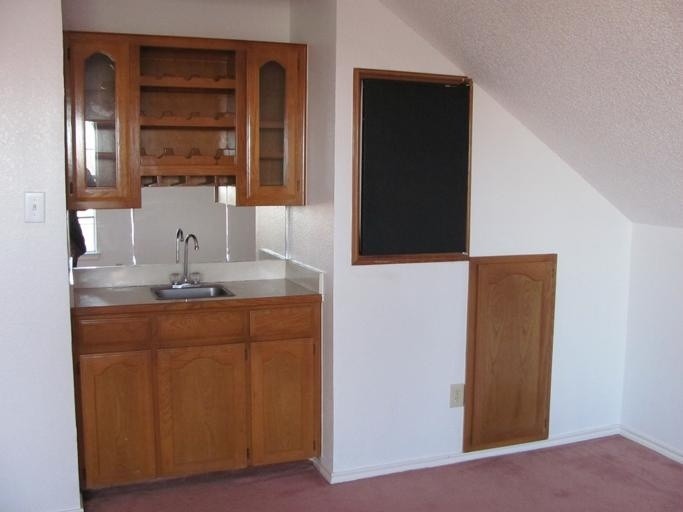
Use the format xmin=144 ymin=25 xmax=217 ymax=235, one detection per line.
xmin=66 ymin=187 xmax=288 ymax=270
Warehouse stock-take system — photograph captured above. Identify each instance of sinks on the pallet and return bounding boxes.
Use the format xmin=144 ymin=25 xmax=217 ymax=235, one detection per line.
xmin=150 ymin=284 xmax=235 ymax=301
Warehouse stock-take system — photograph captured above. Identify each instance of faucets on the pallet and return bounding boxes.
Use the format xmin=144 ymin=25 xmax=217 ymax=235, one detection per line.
xmin=184 ymin=233 xmax=199 ymax=280
xmin=175 ymin=229 xmax=184 ymax=263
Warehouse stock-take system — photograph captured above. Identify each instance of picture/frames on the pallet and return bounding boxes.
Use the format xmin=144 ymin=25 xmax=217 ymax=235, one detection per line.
xmin=354 ymin=65 xmax=474 ymax=261
